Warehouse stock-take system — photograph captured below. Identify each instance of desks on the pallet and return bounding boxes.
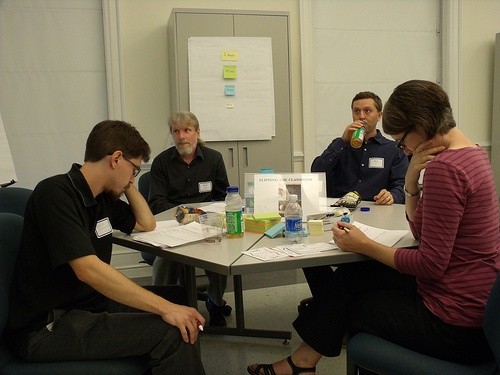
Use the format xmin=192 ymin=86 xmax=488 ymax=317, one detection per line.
xmin=112 ymin=198 xmax=422 ymax=345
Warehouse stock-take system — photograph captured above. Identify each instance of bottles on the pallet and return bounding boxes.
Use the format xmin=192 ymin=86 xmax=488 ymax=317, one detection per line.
xmin=225 ymin=187 xmax=245 ymax=239
xmin=246 ymin=182 xmax=254 ymax=213
xmin=284 ymin=195 xmax=304 ymax=243
xmin=351 ymin=120 xmax=367 ymax=149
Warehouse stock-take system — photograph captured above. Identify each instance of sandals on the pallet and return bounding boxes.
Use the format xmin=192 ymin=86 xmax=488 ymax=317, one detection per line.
xmin=246 ymin=356 xmax=318 ymax=374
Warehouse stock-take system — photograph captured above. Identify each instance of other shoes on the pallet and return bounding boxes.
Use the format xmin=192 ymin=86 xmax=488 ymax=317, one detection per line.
xmin=204 ymin=299 xmax=229 ymax=329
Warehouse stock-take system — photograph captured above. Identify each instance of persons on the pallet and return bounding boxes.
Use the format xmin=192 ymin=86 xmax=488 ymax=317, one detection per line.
xmin=303 ymin=91 xmax=410 ymax=297
xmin=149 ymin=112 xmax=230 ymax=329
xmin=246 ymin=79 xmax=500 ymax=375
xmin=0 ymin=119 xmax=207 ymax=375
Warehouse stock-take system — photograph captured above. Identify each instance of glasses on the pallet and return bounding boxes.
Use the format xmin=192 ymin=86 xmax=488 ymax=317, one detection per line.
xmin=395 ymin=128 xmax=415 ymax=151
xmin=109 ymin=152 xmax=142 ymax=178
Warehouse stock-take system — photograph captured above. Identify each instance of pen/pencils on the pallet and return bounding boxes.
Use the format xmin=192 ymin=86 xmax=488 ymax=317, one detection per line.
xmin=322 ymin=212 xmax=335 ymax=219
xmin=343 ymin=227 xmax=351 ymax=232
xmin=337 ymin=212 xmax=348 ymax=217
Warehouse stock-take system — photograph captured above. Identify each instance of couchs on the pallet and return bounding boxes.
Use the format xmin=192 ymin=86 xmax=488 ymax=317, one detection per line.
xmin=0 ymin=187 xmax=161 ymax=375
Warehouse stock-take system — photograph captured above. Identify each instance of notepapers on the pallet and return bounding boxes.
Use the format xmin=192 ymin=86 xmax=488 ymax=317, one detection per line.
xmin=264 ymin=222 xmax=286 ymax=239
xmin=308 ymin=220 xmax=324 ymax=236
xmin=245 ymin=218 xmax=280 ymax=235
xmin=252 ymin=213 xmax=282 ymax=221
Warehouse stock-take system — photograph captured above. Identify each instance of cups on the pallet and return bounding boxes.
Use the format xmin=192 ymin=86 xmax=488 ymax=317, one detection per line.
xmin=197 ymin=213 xmax=223 ymax=243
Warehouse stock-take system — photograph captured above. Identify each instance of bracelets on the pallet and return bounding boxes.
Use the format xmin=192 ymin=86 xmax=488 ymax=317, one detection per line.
xmin=403 ymin=185 xmax=420 ymax=197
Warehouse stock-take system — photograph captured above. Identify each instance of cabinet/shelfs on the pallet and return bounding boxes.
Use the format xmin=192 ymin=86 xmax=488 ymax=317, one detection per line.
xmin=166 ymin=8 xmax=294 ymax=197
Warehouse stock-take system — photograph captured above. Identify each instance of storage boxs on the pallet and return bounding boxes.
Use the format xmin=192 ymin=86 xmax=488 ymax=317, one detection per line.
xmin=306 ymin=213 xmax=353 ymax=231
xmin=245 ymin=214 xmax=281 ymax=234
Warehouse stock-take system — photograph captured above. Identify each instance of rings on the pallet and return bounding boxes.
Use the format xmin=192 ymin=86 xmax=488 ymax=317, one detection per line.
xmin=388 ymin=197 xmax=391 ymax=200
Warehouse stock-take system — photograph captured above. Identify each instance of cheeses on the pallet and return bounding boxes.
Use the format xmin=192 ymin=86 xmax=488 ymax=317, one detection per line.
xmin=307 ymin=219 xmax=323 ymax=236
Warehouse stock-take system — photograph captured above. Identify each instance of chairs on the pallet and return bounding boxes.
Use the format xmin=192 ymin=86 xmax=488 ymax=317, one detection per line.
xmin=346 ymin=270 xmax=500 ymax=375
